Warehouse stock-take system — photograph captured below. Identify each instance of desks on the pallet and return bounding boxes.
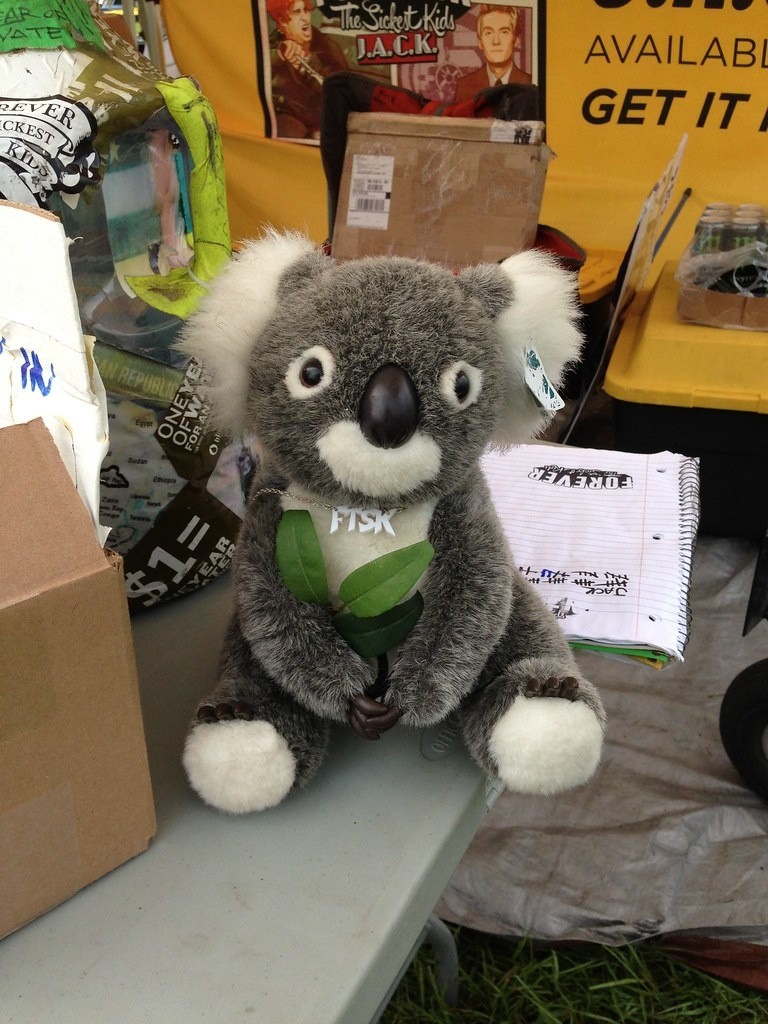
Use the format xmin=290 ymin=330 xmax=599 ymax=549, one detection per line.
xmin=0 ymin=572 xmax=504 ymax=1024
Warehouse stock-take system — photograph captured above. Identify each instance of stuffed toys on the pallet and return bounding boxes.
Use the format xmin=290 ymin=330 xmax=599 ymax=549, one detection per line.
xmin=174 ymin=228 xmax=608 ymax=812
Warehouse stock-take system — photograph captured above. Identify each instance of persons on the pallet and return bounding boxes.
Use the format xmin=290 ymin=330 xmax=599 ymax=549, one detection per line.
xmin=449 ymin=8 xmax=531 ymax=98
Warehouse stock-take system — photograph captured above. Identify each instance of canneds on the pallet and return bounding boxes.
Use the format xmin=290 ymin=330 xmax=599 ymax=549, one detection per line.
xmin=691 ymin=203 xmax=764 ymax=289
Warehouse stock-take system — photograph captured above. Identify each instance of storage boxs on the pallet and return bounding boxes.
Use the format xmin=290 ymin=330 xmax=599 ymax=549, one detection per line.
xmin=331 ymin=111 xmax=553 ymax=276
xmin=1 ymin=417 xmax=159 ymax=943
xmin=601 ymin=261 xmax=768 ymax=548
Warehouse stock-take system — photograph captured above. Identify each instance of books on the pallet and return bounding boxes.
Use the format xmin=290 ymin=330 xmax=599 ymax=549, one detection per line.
xmin=479 ymin=443 xmax=698 ymax=666
